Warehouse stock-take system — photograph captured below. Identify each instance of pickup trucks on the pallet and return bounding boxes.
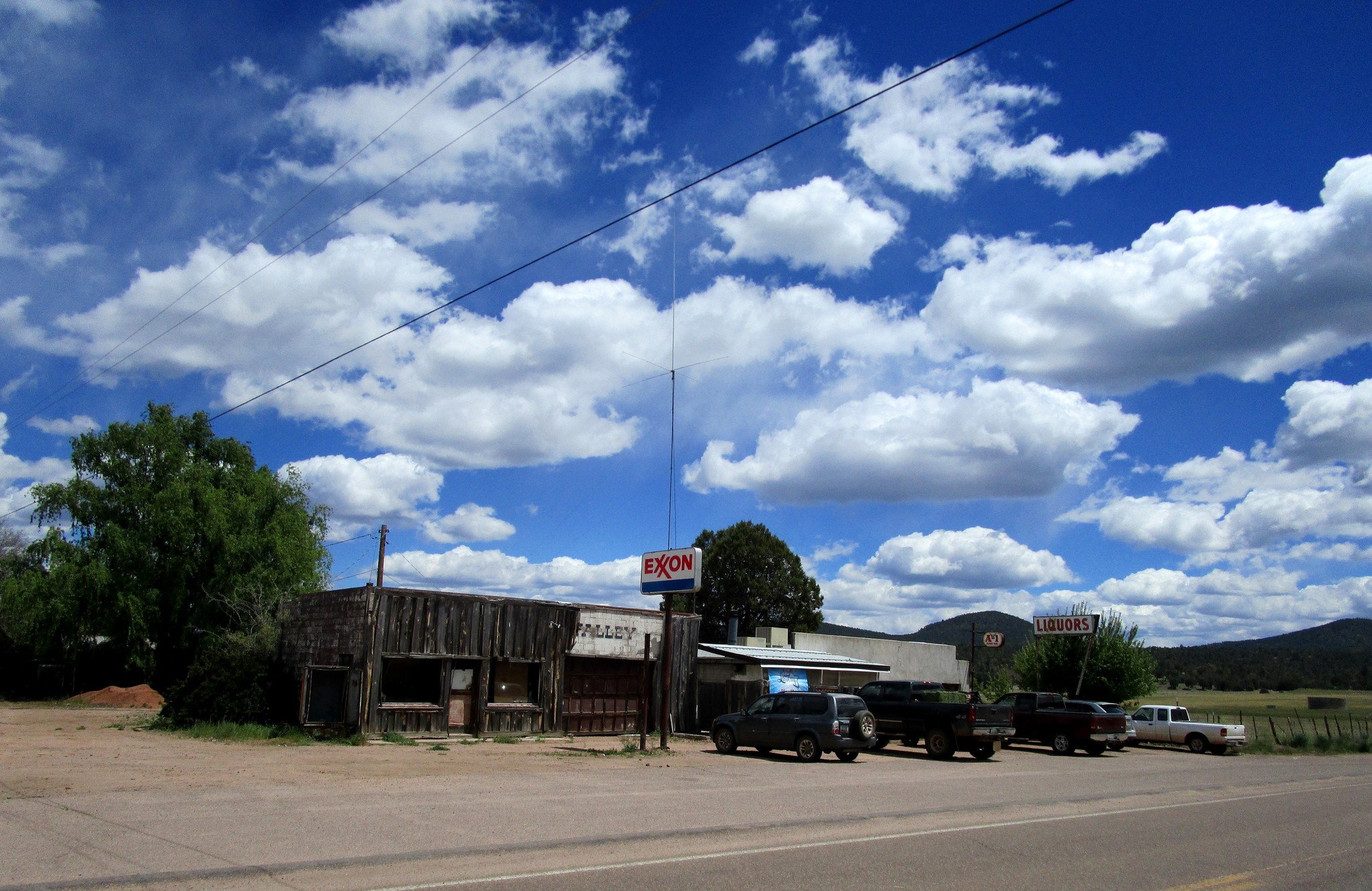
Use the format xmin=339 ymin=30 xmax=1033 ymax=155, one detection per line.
xmin=993 ymin=691 xmax=1128 ymax=758
xmin=1132 ymin=705 xmax=1247 ymax=757
xmin=854 ymin=680 xmax=1017 ymax=760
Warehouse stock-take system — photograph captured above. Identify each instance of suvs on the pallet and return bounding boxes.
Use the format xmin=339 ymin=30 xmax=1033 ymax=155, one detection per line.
xmin=1064 ymin=699 xmax=1136 ymax=751
xmin=710 ymin=690 xmax=877 ymax=762
